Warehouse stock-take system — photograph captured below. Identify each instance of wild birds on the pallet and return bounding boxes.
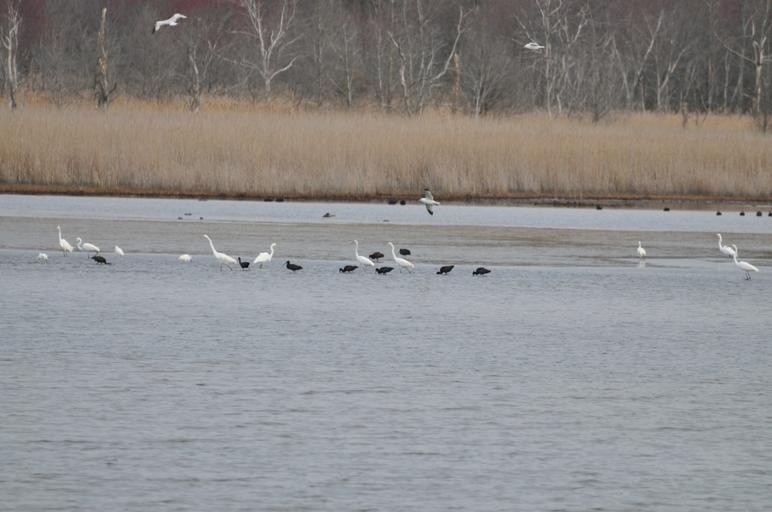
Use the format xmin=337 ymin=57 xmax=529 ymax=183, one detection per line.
xmin=436 ymin=263 xmax=456 ymax=275
xmin=715 ymin=233 xmax=735 ymax=258
xmin=339 ymin=239 xmax=415 ymax=276
xmin=472 ymin=266 xmax=492 ymax=275
xmin=416 ymin=187 xmax=438 ymax=217
xmin=151 ymin=11 xmax=188 ymax=35
xmin=635 ymin=240 xmax=648 ymax=260
xmin=729 ymin=244 xmax=758 ymax=281
xmin=177 ymin=254 xmax=193 ymax=263
xmin=522 ymin=39 xmax=545 ymax=52
xmin=37 ymin=224 xmax=125 ymax=265
xmin=203 ymin=232 xmax=304 ymax=273
